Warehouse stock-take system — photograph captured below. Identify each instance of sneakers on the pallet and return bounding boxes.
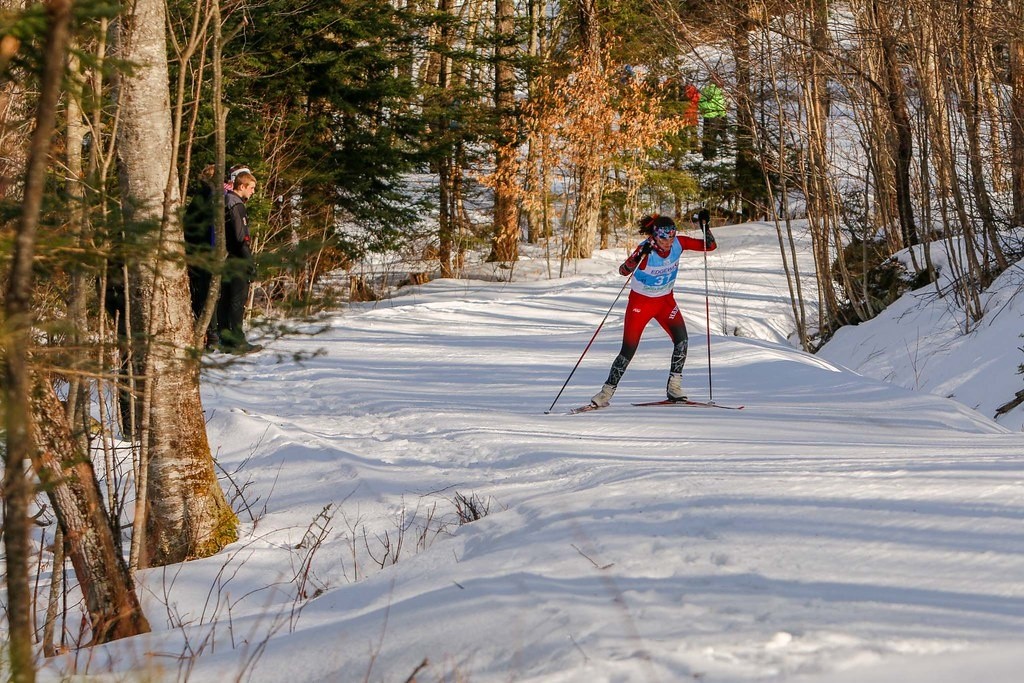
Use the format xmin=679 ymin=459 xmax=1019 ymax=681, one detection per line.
xmin=666 ymin=375 xmax=688 ymax=401
xmin=590 ymin=384 xmax=616 ymax=406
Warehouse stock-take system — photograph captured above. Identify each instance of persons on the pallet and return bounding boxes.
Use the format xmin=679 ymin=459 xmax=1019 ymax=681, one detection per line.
xmin=591 ymin=208 xmax=717 ymax=408
xmin=184 ymin=164 xmax=263 ymax=355
xmin=96 ymin=230 xmax=145 ymax=442
xmin=619 ymin=65 xmax=731 ymax=161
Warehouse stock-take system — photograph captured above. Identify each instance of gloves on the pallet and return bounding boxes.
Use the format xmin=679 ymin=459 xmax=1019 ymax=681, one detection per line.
xmin=698 ymin=209 xmax=710 ymax=231
xmin=635 ymin=242 xmax=653 ymax=263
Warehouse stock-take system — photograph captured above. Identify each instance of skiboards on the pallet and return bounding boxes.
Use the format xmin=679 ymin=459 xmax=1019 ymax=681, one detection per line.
xmin=569 ymin=398 xmax=745 ymax=414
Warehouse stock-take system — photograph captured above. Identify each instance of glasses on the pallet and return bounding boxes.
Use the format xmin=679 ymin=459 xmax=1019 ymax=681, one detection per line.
xmin=659 ymin=238 xmax=675 ymax=244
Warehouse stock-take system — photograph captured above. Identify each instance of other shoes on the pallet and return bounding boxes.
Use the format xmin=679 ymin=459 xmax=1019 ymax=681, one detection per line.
xmin=234 ymin=342 xmax=262 ymax=353
